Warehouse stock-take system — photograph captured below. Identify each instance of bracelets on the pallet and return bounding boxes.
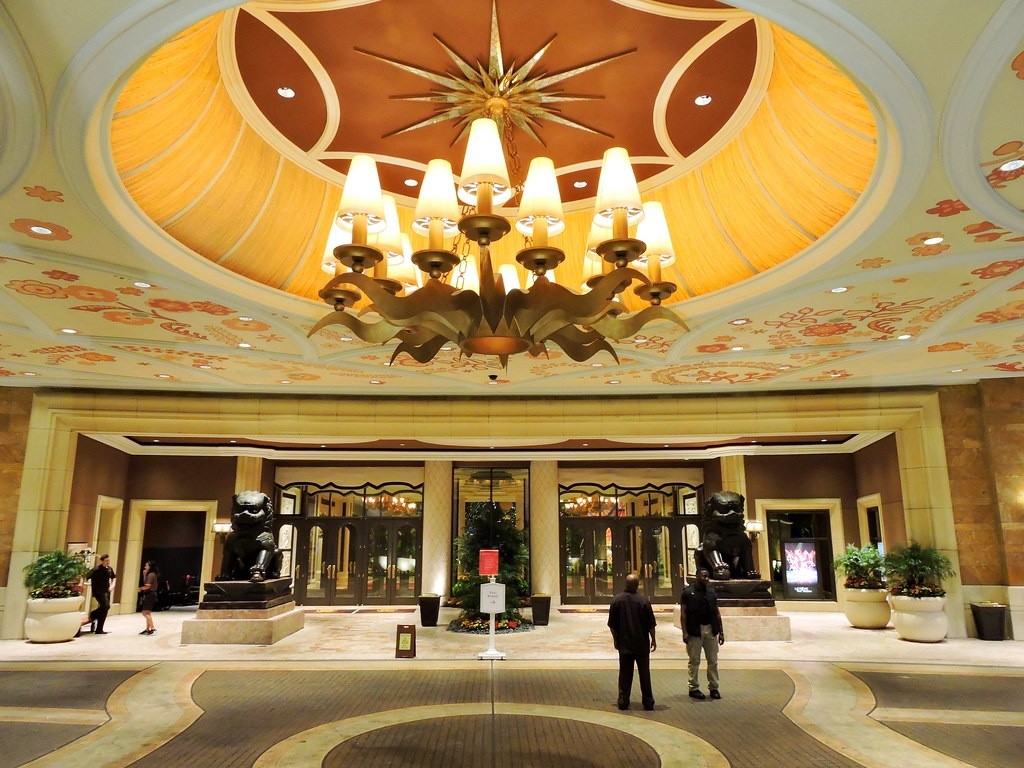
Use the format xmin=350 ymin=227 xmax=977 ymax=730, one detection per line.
xmin=682 ymin=627 xmax=687 ymax=632
xmin=720 ymin=631 xmax=723 ymax=635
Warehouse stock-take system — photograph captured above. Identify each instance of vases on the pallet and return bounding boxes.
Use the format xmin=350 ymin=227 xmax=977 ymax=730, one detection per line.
xmin=419 ymin=593 xmax=441 ymax=626
xmin=970 ymin=602 xmax=1006 ymax=641
xmin=532 ymin=595 xmax=551 ymax=626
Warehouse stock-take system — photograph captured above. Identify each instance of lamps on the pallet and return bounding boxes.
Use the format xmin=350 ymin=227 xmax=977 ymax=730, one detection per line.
xmin=303 ymin=0 xmax=693 ymax=366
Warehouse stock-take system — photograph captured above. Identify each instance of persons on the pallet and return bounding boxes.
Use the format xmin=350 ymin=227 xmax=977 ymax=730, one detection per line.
xmin=680 ymin=568 xmax=725 ymax=700
xmin=607 ymin=574 xmax=657 ymax=710
xmin=137 ymin=561 xmax=158 ymax=635
xmin=79 ymin=553 xmax=116 ymax=634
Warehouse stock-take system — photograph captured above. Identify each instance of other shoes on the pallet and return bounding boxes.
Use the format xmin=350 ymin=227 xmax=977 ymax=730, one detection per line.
xmin=710 ymin=689 xmax=720 ymax=699
xmin=643 ymin=705 xmax=654 ymax=710
xmin=689 ymin=690 xmax=705 ymax=700
xmin=617 ymin=704 xmax=628 ymax=710
xmin=139 ymin=629 xmax=155 ymax=635
xmin=95 ymin=631 xmax=107 ymax=634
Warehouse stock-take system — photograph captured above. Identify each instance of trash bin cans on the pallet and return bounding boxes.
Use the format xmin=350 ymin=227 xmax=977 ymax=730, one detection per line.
xmin=531 ymin=594 xmax=551 ymax=625
xmin=970 ymin=600 xmax=1007 ymax=640
xmin=418 ymin=593 xmax=441 ymax=626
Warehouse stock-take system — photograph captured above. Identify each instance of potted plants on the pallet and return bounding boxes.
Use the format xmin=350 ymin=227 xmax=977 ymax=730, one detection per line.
xmin=831 ymin=543 xmax=891 ymax=629
xmin=22 ymin=549 xmax=90 ymax=643
xmin=883 ymin=540 xmax=957 ymax=643
